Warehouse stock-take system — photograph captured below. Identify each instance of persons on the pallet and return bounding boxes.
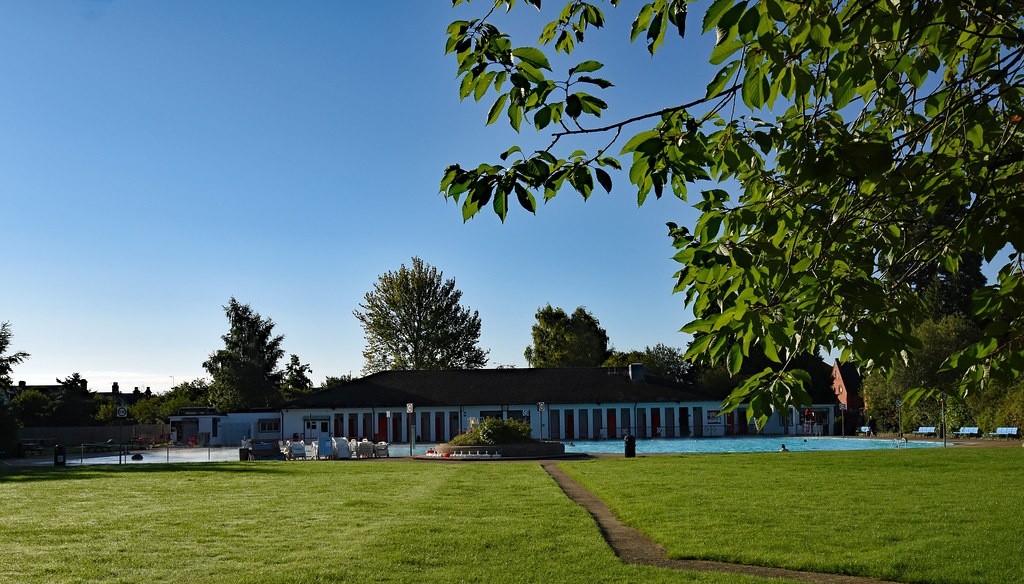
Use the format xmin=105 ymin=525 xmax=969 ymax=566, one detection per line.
xmin=779 ymin=444 xmax=789 ymax=451
xmin=569 ymin=441 xmax=575 ymax=446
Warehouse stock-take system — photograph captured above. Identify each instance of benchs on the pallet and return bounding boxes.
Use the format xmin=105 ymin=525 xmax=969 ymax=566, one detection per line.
xmin=989 ymin=427 xmax=1019 ymax=440
xmin=856 ymin=426 xmax=870 ymax=436
xmin=913 ymin=427 xmax=936 ymax=438
xmin=953 ymin=427 xmax=979 ymax=438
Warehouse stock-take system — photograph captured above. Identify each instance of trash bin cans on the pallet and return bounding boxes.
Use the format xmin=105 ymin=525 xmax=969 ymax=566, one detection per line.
xmin=624 ymin=435 xmax=635 ymax=458
xmin=239 ymin=448 xmax=249 ymax=461
xmin=53 ymin=444 xmax=65 ymax=467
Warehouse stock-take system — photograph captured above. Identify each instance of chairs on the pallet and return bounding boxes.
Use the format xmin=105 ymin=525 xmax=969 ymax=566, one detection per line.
xmin=249 ymin=434 xmax=389 ymax=461
